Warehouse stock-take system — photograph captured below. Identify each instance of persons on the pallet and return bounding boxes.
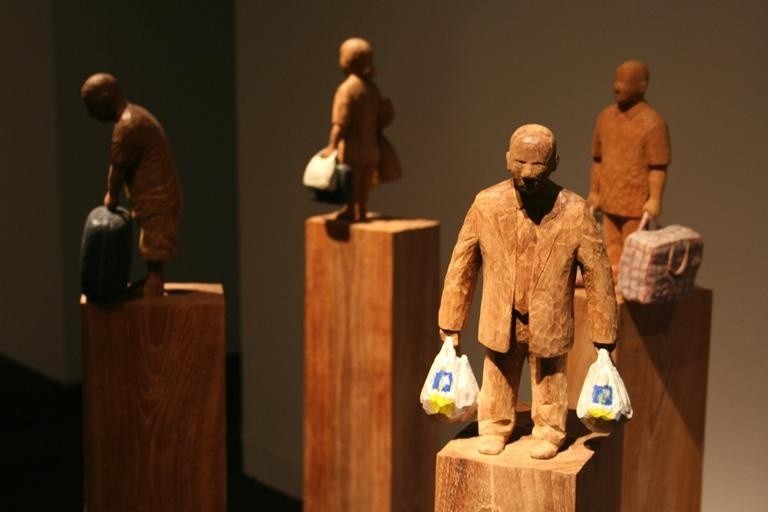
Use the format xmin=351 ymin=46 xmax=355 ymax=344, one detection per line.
xmin=320 ymin=37 xmax=381 ymax=224
xmin=586 ymin=59 xmax=672 ymax=290
xmin=437 ymin=122 xmax=618 ymax=460
xmin=83 ymin=72 xmax=183 ymax=298
